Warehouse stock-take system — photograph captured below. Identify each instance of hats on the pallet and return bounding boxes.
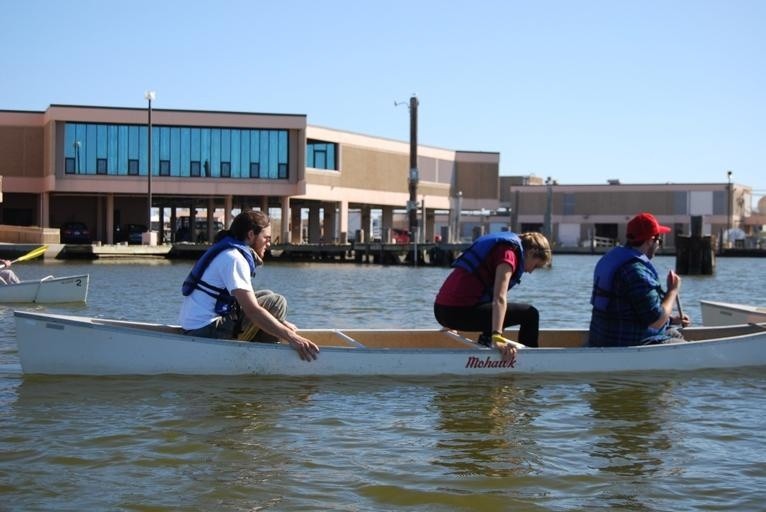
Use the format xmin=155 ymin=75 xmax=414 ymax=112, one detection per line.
xmin=626 ymin=214 xmax=679 ymax=239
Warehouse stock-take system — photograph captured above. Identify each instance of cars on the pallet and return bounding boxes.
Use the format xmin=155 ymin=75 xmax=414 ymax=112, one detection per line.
xmin=55 ymin=216 xmax=223 ymax=244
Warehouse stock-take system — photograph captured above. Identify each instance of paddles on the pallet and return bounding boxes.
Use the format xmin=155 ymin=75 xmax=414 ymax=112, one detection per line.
xmin=0 ymin=244 xmax=49 ymax=270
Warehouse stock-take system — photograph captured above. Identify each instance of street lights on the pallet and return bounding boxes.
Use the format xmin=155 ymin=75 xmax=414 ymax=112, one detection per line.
xmin=73 ymin=142 xmax=81 ymax=174
xmin=144 ymin=90 xmax=155 ymax=232
xmin=456 ymin=191 xmax=462 ymax=242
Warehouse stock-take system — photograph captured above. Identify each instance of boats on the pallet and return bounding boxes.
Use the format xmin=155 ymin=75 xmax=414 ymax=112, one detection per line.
xmin=13 ymin=310 xmax=766 ymax=375
xmin=697 ymin=300 xmax=766 ymax=327
xmin=0 ymin=273 xmax=89 ymax=303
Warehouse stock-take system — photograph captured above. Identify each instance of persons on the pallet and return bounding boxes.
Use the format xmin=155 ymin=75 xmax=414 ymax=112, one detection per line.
xmin=176 ymin=209 xmax=320 ymax=363
xmin=586 ymin=210 xmax=692 ymax=347
xmin=0 ymin=257 xmax=22 ymax=286
xmin=432 ymin=228 xmax=554 ymax=364
